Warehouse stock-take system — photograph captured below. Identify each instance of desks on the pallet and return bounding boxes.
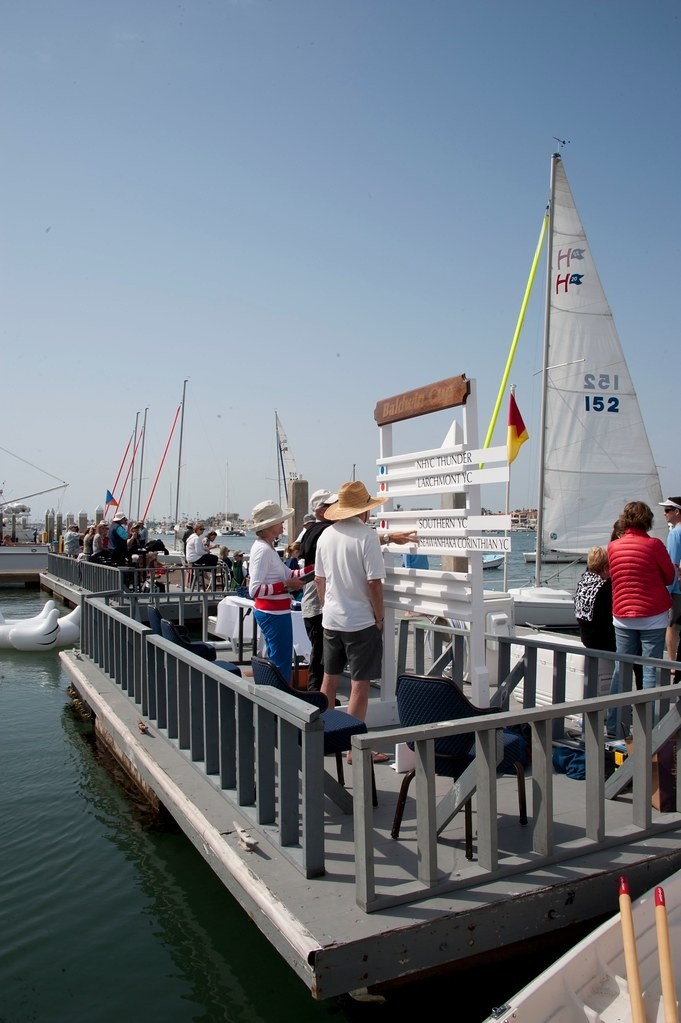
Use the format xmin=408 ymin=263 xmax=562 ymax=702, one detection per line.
xmin=214 ymin=595 xmax=343 ymax=707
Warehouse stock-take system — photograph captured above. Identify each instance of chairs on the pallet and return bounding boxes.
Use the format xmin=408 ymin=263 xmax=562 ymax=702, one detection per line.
xmin=148 ymin=603 xmax=242 ymax=676
xmin=393 ymin=673 xmax=528 ymax=859
xmin=216 ymin=559 xmax=236 ymax=589
xmin=251 ymin=656 xmax=378 ymax=807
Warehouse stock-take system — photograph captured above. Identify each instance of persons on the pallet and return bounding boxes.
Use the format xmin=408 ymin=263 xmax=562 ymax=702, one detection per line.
xmin=403 ymin=553 xmax=430 ymax=618
xmin=575 ymin=496 xmax=681 ymax=739
xmin=247 ymin=501 xmax=315 ymax=683
xmin=32 ymin=529 xmax=39 ymax=542
xmin=3 ymin=535 xmax=15 ymax=546
xmin=286 ymin=482 xmax=420 ymax=764
xmin=175 ymin=520 xmax=249 ymax=585
xmin=64 ymin=513 xmax=166 ymax=593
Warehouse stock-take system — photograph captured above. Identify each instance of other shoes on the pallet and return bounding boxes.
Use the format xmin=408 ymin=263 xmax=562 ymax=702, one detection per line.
xmin=607 ymin=734 xmax=631 ymax=740
xmin=347 ymin=752 xmax=390 ymax=765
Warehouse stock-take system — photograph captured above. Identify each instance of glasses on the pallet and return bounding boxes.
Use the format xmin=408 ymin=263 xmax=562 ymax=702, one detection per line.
xmin=200 ymin=528 xmax=205 ymax=530
xmin=665 ymin=508 xmax=677 ymax=513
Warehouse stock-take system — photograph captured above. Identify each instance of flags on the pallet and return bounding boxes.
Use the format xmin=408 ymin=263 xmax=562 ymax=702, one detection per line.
xmin=507 ymin=395 xmax=529 ymax=464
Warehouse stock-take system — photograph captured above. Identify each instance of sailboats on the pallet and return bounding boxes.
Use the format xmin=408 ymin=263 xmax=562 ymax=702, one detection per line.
xmin=507 ymin=153 xmax=671 ymax=625
xmin=129 ymin=380 xmax=250 ymax=565
xmin=275 ymin=410 xmax=301 ymax=547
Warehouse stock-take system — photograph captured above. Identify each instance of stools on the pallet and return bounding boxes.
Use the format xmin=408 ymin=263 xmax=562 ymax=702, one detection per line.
xmin=189 ymin=563 xmax=208 ymax=601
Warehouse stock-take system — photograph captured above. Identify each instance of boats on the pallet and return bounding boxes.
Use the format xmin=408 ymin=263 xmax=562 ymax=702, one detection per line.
xmin=482 ymin=554 xmax=504 ymax=570
xmin=523 ymin=551 xmax=587 ymax=563
xmin=216 ymin=525 xmax=246 ymax=537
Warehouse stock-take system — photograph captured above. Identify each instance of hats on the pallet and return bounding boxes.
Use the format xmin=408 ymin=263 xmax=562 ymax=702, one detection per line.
xmin=248 ymin=500 xmax=295 ymax=533
xmin=130 ymin=523 xmax=140 ymax=531
xmin=100 ymin=520 xmax=108 ymax=525
xmin=233 ymin=550 xmax=245 ymax=557
xmin=69 ymin=524 xmax=77 ymax=529
xmin=658 ymin=499 xmax=681 ymax=509
xmin=113 ymin=512 xmax=126 ymax=521
xmin=303 ymin=514 xmax=316 ymax=525
xmin=309 ymin=489 xmax=338 ymax=512
xmin=324 ymin=480 xmax=388 ymax=521
xmin=186 ymin=521 xmax=193 ymax=527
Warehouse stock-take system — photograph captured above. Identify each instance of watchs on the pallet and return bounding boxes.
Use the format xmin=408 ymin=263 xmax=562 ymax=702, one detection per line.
xmin=383 ymin=533 xmax=389 ymax=544
